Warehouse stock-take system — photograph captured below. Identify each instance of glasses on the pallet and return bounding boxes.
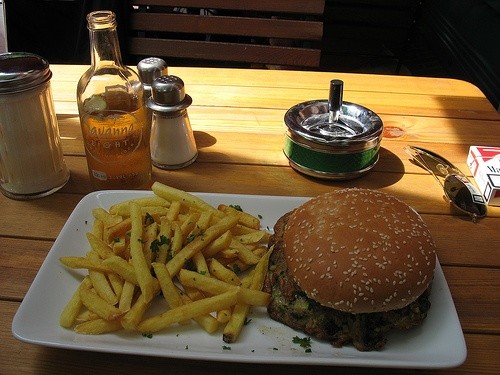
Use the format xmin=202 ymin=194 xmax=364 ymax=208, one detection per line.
xmin=403 ymin=144 xmax=488 ymax=225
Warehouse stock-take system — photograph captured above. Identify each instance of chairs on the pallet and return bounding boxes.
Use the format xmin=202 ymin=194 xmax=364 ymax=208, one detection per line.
xmin=130 ymin=0 xmax=327 ymax=71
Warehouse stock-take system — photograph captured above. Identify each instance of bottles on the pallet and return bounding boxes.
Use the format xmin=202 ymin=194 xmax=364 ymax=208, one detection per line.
xmin=0 ymin=53 xmax=70 ymax=200
xmin=146 ymin=74 xmax=198 ymax=171
xmin=137 ymin=57 xmax=168 ymax=134
xmin=77 ymin=11 xmax=154 ymax=191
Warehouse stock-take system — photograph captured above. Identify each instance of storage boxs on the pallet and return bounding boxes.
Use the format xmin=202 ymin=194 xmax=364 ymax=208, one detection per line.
xmin=467 ymin=145 xmax=500 ymax=206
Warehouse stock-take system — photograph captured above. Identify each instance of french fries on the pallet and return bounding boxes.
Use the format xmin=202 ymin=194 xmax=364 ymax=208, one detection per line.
xmin=58 ymin=181 xmax=275 ymax=344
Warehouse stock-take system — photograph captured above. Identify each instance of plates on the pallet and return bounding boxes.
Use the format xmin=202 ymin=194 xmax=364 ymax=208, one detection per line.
xmin=11 ymin=190 xmax=467 ymax=368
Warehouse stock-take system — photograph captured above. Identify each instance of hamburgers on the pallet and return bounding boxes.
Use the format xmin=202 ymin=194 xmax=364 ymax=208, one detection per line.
xmin=263 ymin=187 xmax=438 ymax=352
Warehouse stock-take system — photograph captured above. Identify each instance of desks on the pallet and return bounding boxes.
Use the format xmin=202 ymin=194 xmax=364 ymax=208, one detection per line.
xmin=0 ymin=64 xmax=500 ymax=375
